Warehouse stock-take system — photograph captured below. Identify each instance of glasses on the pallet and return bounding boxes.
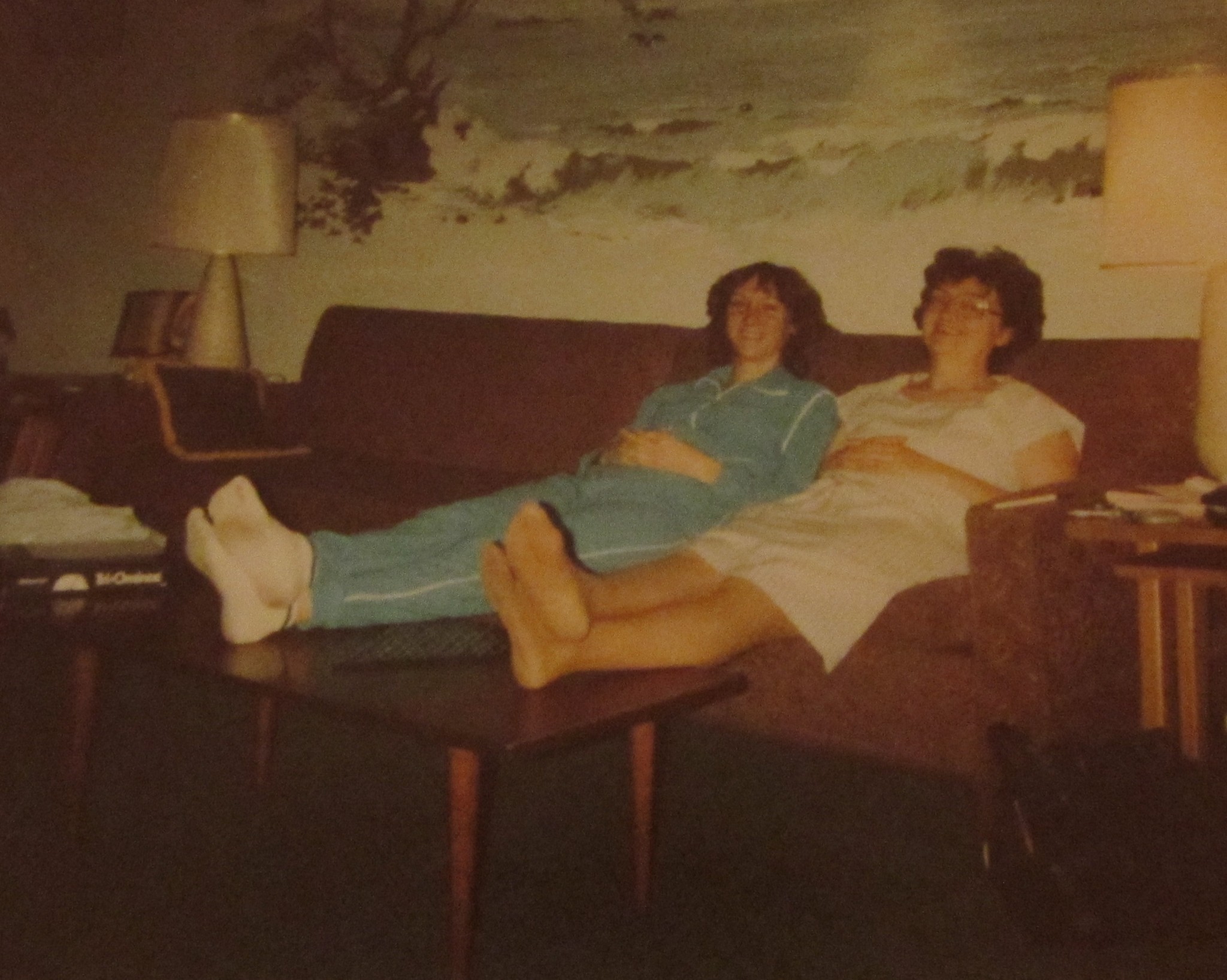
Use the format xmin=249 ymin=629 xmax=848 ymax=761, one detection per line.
xmin=928 ymin=288 xmax=1004 ymax=320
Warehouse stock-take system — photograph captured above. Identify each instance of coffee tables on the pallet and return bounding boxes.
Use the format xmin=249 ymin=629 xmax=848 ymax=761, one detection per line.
xmin=0 ymin=551 xmax=749 ymax=980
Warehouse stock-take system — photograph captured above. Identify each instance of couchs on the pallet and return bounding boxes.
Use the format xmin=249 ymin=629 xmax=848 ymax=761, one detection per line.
xmin=104 ymin=302 xmax=1200 ymax=877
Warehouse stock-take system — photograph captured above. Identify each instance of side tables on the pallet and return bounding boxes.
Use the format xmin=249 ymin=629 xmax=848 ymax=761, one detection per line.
xmin=1062 ymin=494 xmax=1227 ymax=765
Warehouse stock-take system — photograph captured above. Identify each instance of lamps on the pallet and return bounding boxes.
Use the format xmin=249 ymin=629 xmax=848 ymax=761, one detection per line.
xmin=145 ymin=112 xmax=298 ymax=370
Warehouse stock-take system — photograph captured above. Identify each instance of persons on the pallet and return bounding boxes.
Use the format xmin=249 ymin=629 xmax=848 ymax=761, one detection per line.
xmin=185 ymin=263 xmax=836 ymax=647
xmin=484 ymin=246 xmax=1088 ymax=692
